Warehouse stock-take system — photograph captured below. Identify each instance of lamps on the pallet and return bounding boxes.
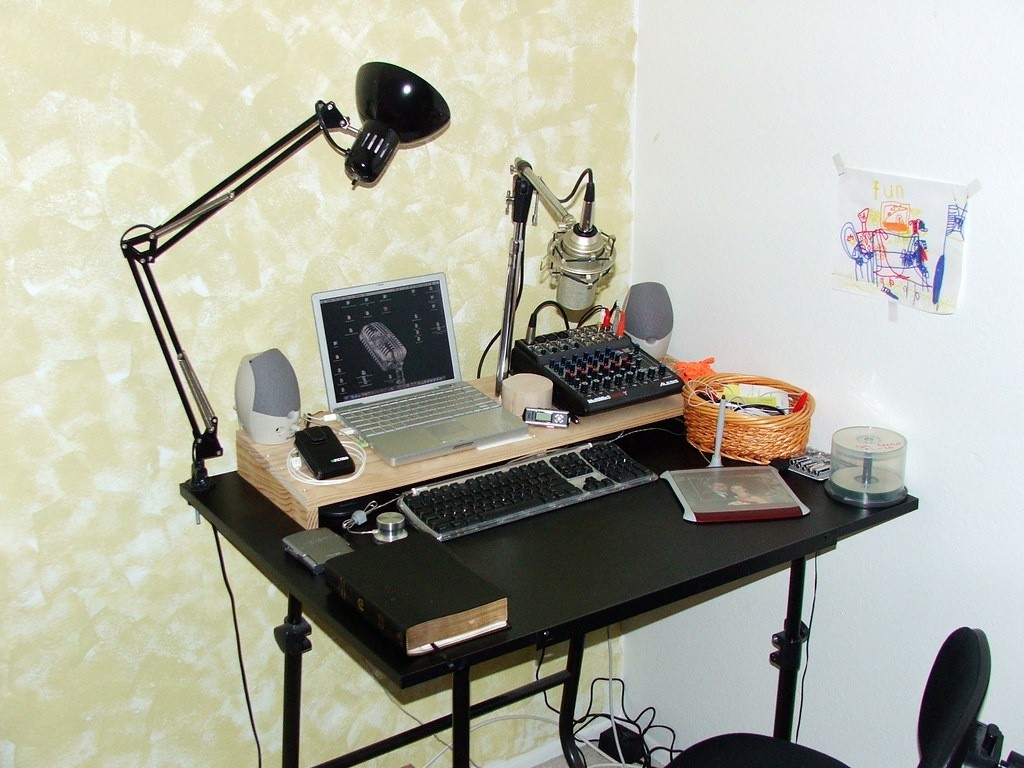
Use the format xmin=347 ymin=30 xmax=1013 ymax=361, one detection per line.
xmin=494 ymin=155 xmax=618 ymax=395
xmin=118 ymin=60 xmax=450 ymax=492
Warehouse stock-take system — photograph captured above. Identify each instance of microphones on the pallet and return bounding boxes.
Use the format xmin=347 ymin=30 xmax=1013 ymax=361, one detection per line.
xmin=555 ymin=182 xmax=605 ymax=310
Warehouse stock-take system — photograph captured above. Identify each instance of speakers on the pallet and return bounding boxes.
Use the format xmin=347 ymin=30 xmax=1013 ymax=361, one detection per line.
xmin=234 ymin=348 xmax=301 ymax=444
xmin=620 ymin=282 xmax=674 ymax=364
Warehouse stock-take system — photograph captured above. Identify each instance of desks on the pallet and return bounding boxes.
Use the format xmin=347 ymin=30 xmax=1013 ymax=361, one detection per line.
xmin=178 ymin=416 xmax=918 ymax=768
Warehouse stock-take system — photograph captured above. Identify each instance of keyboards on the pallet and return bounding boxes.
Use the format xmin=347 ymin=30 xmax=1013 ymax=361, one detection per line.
xmin=396 ymin=440 xmax=659 ymax=543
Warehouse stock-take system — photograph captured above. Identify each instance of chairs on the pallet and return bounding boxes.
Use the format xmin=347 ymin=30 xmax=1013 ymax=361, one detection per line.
xmin=665 ymin=627 xmax=1024 ymax=768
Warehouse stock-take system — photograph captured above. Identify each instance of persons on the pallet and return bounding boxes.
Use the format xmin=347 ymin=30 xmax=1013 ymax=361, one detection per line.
xmin=700 ymin=482 xmax=767 ymax=507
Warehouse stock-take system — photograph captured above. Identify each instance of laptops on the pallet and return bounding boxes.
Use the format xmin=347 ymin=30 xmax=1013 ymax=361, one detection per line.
xmin=311 ymin=272 xmax=529 ymax=468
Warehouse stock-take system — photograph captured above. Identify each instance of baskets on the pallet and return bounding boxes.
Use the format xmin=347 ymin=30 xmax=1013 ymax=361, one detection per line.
xmin=681 ymin=372 xmax=815 ymax=460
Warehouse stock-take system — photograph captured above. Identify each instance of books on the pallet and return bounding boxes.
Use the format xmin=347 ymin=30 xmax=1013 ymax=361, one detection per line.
xmin=325 ymin=532 xmax=509 ymax=657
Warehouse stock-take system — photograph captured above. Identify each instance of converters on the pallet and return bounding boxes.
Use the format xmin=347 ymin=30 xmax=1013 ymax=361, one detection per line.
xmin=597 ymin=724 xmax=645 ymax=764
